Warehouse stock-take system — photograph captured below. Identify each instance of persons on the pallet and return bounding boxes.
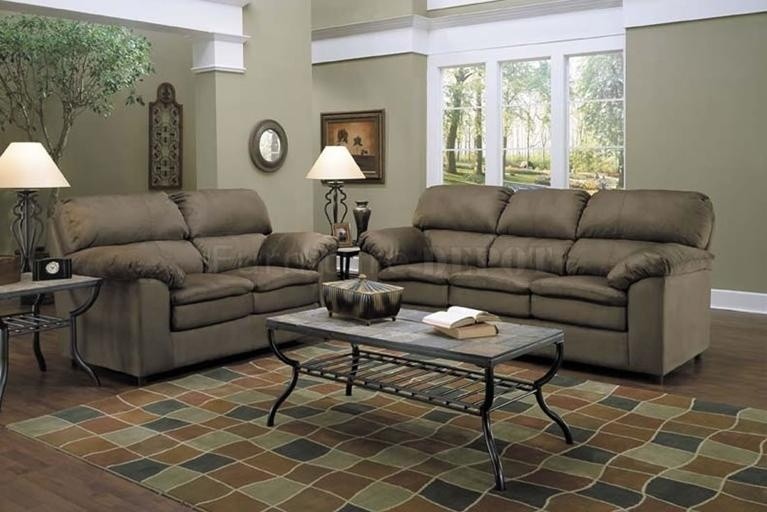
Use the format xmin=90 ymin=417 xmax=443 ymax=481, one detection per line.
xmin=338 ymin=232 xmax=345 ymax=240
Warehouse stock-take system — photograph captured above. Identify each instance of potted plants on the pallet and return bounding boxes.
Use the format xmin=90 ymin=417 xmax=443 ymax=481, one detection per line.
xmin=0 ymin=11 xmax=155 ymax=307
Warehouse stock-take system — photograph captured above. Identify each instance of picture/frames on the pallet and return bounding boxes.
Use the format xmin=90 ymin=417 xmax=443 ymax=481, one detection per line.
xmin=331 ymin=222 xmax=353 ymax=248
xmin=319 ymin=110 xmax=385 ymax=186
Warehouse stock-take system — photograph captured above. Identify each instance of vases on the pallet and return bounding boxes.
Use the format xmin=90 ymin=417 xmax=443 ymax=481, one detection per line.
xmin=353 ymin=200 xmax=372 ymax=242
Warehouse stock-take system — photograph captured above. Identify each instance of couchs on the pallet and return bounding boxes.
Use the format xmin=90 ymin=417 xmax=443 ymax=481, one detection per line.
xmin=355 ymin=184 xmax=717 ymax=384
xmin=46 ymin=186 xmax=339 ymax=389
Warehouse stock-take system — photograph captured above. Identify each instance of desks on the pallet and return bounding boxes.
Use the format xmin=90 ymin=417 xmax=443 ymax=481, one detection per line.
xmin=0 ymin=273 xmax=104 ymax=405
xmin=333 ymin=244 xmax=362 ymax=282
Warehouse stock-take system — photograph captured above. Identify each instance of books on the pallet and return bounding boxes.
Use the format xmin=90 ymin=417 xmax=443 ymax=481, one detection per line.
xmin=431 ymin=321 xmax=498 ymax=339
xmin=421 ymin=306 xmax=501 ymax=330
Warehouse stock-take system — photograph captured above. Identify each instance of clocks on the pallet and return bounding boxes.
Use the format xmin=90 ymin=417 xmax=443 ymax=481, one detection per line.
xmin=32 ymin=257 xmax=73 ymax=280
xmin=249 ymin=120 xmax=288 ymax=173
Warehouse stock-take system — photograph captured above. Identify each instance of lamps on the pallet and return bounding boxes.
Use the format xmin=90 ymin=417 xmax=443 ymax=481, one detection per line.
xmin=0 ymin=142 xmax=72 ymax=272
xmin=302 ymin=145 xmax=366 ymax=238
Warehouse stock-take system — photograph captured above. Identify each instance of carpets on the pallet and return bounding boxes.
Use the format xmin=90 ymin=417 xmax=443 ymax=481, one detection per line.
xmin=4 ymin=341 xmax=767 ymax=512
